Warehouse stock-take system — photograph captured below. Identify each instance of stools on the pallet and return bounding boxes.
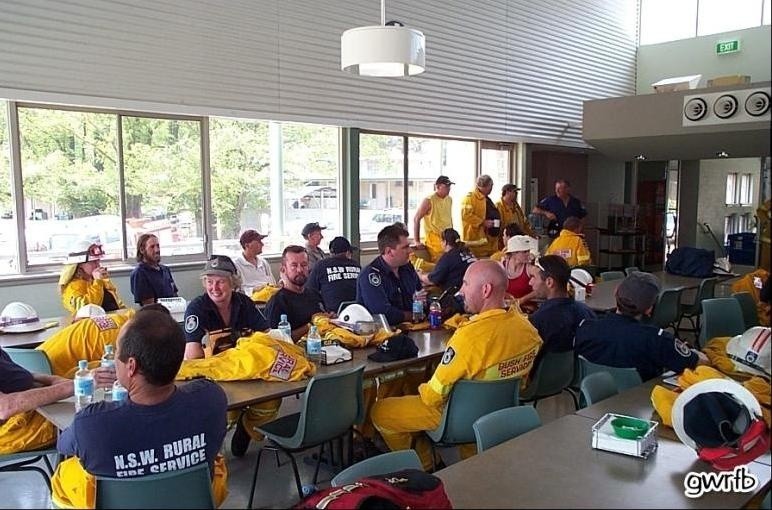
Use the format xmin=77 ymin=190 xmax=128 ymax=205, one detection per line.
xmin=0 ymin=447 xmax=60 ymax=499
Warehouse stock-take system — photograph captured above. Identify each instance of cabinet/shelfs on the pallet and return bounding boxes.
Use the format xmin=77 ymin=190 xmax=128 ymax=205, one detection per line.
xmin=598 ymin=229 xmax=647 ymax=273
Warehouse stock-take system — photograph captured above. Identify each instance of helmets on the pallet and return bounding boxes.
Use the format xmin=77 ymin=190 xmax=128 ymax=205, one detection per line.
xmin=73 ymin=303 xmax=105 ymax=320
xmin=0 ymin=303 xmax=59 ymax=333
xmin=504 ymin=235 xmax=530 ymax=253
xmin=671 ymin=378 xmax=771 ymax=472
xmin=64 ymin=241 xmax=105 ymax=264
xmin=569 ymin=269 xmax=593 ymax=296
xmin=330 ymin=304 xmax=377 ymax=335
xmin=727 ymin=327 xmax=771 ymax=378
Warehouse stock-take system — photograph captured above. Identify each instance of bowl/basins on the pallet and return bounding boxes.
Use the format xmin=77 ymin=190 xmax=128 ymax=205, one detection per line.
xmin=611 ymin=417 xmax=649 ymax=438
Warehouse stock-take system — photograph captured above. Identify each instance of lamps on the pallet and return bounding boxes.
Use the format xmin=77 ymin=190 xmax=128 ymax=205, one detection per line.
xmin=337 ymin=2 xmax=429 ymax=83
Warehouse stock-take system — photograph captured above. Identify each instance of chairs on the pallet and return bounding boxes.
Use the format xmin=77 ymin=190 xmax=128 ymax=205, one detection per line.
xmin=91 ymin=462 xmax=218 ymax=509
xmin=0 ymin=348 xmax=54 ymax=377
xmin=247 ymin=364 xmax=368 ymax=510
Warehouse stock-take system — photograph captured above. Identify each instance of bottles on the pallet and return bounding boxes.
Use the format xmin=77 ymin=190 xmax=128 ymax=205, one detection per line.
xmin=428 ymin=296 xmax=441 ymax=329
xmin=102 ymin=345 xmax=116 ymax=395
xmin=277 ymin=314 xmax=292 ymax=339
xmin=307 ymin=327 xmax=322 ymax=365
xmin=74 ymin=359 xmax=95 ymax=414
xmin=413 ymin=287 xmax=423 ymax=323
xmin=111 ymin=378 xmax=128 ymax=403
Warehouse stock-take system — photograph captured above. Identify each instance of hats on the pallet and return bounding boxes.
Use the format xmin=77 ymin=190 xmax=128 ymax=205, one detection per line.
xmin=200 ymin=223 xmax=358 ymax=277
xmin=369 ymin=335 xmax=418 ymax=361
xmin=503 ymin=184 xmax=520 ymax=191
xmin=548 ymin=221 xmax=559 ymax=237
xmin=437 ymin=177 xmax=455 ymax=185
xmin=616 ymin=270 xmax=661 ymax=311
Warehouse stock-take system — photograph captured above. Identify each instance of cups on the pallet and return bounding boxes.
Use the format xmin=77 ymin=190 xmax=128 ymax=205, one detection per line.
xmin=574 ymin=286 xmax=586 ymax=300
xmin=244 ymin=288 xmax=254 ymax=297
xmin=493 ymin=220 xmax=500 ymax=227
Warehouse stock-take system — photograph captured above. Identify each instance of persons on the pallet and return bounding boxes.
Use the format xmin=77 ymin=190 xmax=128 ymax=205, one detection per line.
xmin=573 ymin=271 xmax=709 ymax=382
xmin=1 ymin=347 xmax=118 ymax=453
xmin=53 ymin=311 xmax=229 ymax=509
xmin=55 ymin=176 xmax=595 ymax=474
xmin=33 ymin=304 xmax=169 ymax=378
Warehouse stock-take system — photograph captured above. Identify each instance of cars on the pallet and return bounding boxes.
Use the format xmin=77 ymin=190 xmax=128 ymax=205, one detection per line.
xmin=362 ymin=208 xmax=404 ymax=235
xmin=55 ymin=211 xmax=73 ymax=220
xmin=31 ymin=209 xmax=47 ymax=220
xmin=360 ymin=197 xmax=369 ymax=209
xmin=1 ymin=210 xmax=13 ymax=219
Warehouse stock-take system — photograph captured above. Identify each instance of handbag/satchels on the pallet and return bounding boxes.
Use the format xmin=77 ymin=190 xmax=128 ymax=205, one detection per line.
xmin=666 ymin=247 xmax=713 ymax=278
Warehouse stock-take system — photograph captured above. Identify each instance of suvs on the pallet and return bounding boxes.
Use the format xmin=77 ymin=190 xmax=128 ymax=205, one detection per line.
xmin=27 ymin=215 xmax=135 ymax=265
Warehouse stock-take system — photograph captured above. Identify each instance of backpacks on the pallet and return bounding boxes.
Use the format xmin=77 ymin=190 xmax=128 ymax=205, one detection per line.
xmin=291 ymin=469 xmax=452 ymax=510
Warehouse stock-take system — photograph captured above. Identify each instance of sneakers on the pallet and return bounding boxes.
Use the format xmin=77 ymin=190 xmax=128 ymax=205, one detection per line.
xmin=232 ymin=411 xmax=251 ymax=456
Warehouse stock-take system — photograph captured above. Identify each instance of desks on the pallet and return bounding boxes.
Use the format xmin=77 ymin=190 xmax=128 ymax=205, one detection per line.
xmin=0 ymin=296 xmax=266 ymax=349
xmin=35 ymin=327 xmax=383 ymax=431
xmin=572 ymin=367 xmax=772 ymax=469
xmin=431 ymin=371 xmax=772 ymax=510
